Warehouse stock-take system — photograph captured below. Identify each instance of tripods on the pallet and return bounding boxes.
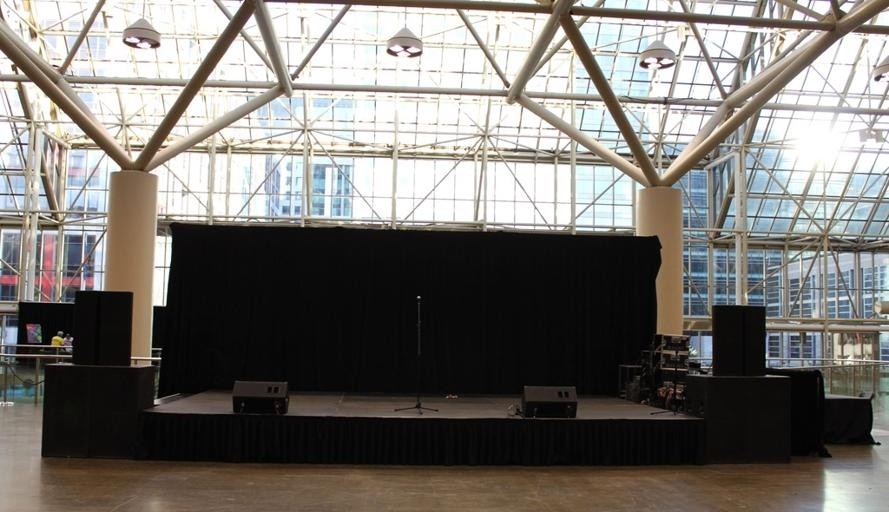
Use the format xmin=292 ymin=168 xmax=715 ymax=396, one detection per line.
xmin=394 ymin=295 xmax=438 ymax=415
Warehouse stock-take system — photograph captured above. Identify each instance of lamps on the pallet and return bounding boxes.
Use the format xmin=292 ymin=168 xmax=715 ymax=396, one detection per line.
xmin=636 ymin=1 xmax=676 ymax=71
xmin=385 ymin=6 xmax=423 ymax=58
xmin=121 ymin=2 xmax=161 ymax=50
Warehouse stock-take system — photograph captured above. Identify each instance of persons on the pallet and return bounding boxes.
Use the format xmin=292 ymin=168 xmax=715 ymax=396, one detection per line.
xmin=50 ymin=330 xmax=65 ymax=352
xmin=64 ymin=333 xmax=73 ymax=351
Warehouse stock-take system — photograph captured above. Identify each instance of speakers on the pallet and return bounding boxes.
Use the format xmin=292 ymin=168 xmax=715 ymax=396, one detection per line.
xmin=74 ymin=289 xmax=133 ymax=367
xmin=232 ymin=380 xmax=292 ymax=413
xmin=712 ymin=304 xmax=768 ymax=377
xmin=522 ymin=384 xmax=578 ymax=416
